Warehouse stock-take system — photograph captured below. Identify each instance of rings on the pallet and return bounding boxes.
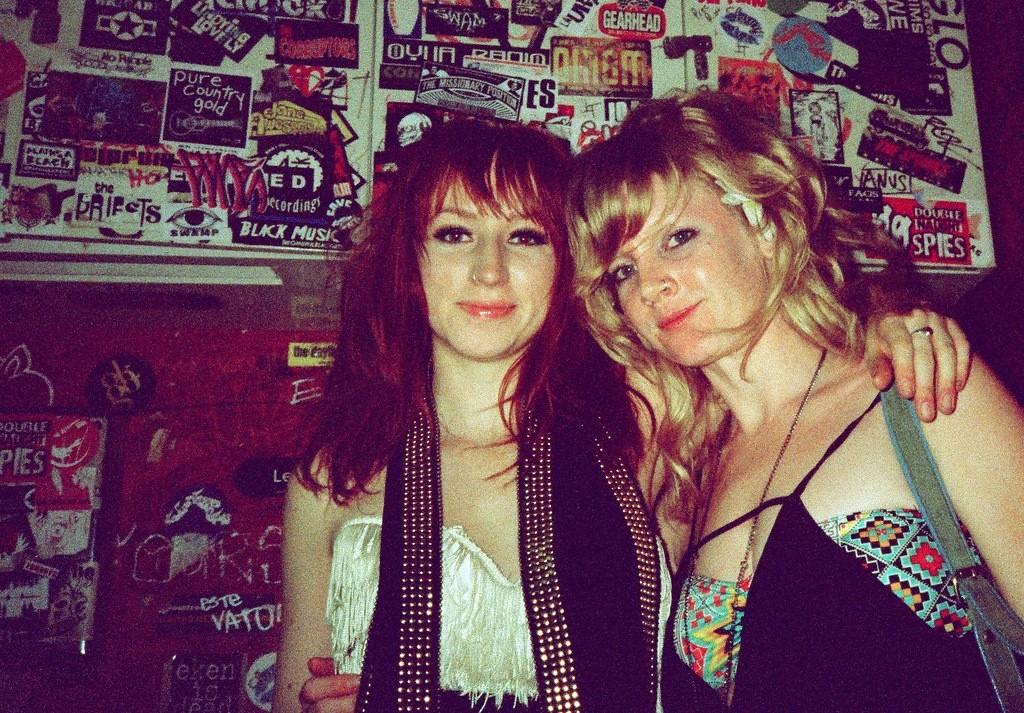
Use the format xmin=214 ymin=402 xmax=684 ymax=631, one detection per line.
xmin=910 ymin=326 xmax=932 ymax=337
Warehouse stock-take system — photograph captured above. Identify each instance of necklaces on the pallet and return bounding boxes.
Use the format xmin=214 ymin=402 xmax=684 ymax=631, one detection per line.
xmin=684 ymin=348 xmax=828 ymax=713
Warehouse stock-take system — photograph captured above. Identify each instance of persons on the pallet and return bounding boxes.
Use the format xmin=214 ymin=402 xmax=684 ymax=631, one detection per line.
xmin=299 ymin=91 xmax=1024 ymax=713
xmin=268 ymin=119 xmax=972 ymax=712
